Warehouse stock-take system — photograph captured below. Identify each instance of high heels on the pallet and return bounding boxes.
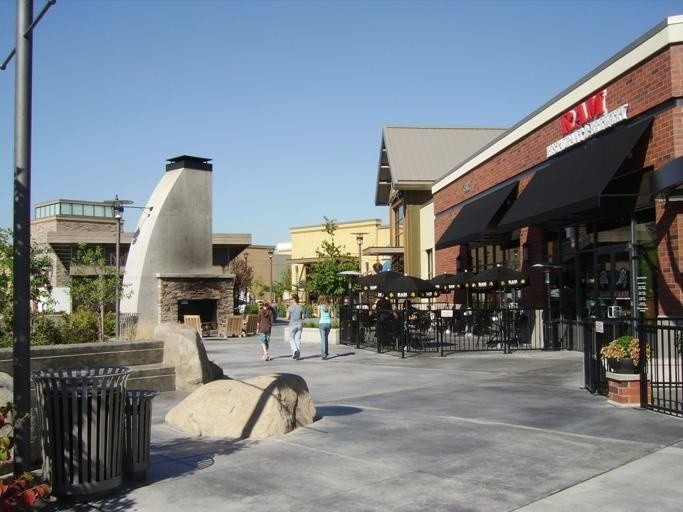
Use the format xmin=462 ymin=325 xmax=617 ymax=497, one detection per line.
xmin=322 ymin=354 xmax=328 ymax=360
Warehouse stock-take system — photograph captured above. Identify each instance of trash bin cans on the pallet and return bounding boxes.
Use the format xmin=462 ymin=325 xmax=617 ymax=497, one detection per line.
xmin=32 ymin=367 xmax=159 ymax=503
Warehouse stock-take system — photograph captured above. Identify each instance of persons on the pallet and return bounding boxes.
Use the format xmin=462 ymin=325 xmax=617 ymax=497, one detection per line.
xmin=312 ymin=293 xmax=332 ymax=360
xmin=286 ymin=294 xmax=304 ymax=358
xmin=256 ymin=299 xmax=273 ymax=361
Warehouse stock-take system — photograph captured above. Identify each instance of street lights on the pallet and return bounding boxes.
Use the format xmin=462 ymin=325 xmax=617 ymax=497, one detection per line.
xmin=268 ymin=249 xmax=274 ymax=303
xmin=357 ymin=236 xmax=364 ymax=312
xmin=104 ymin=195 xmax=133 ymax=340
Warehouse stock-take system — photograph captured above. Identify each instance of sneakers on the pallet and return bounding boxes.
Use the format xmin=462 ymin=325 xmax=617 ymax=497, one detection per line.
xmin=292 ymin=350 xmax=300 ymax=360
xmin=263 ymin=354 xmax=271 ymax=361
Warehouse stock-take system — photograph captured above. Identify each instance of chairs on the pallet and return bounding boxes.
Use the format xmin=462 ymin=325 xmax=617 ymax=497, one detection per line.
xmin=242 ymin=314 xmax=257 ymax=337
xmin=217 ymin=314 xmax=243 ymax=339
xmin=183 ymin=315 xmax=211 ymax=338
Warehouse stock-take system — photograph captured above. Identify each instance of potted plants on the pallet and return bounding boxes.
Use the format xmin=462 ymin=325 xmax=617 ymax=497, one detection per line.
xmin=600 ymin=336 xmax=654 ymax=373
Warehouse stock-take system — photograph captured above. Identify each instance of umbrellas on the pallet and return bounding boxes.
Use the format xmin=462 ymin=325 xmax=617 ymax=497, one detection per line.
xmin=337 ymin=267 xmax=527 ymax=307
